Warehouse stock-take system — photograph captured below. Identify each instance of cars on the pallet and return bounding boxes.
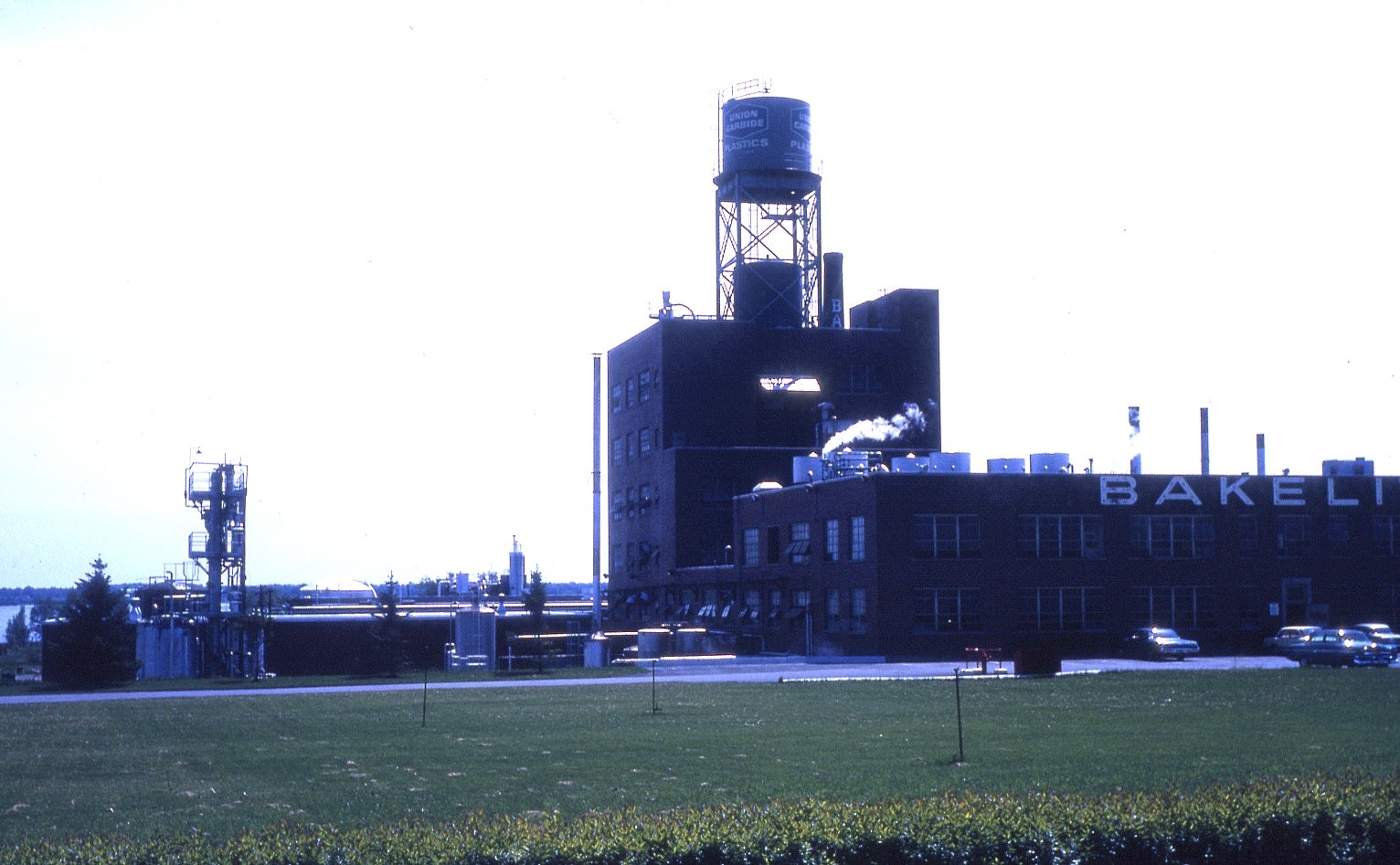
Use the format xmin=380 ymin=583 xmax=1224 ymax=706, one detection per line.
xmin=1353 ymin=623 xmax=1400 ymax=657
xmin=1288 ymin=629 xmax=1395 ymax=671
xmin=1264 ymin=626 xmax=1322 ymax=655
xmin=1120 ymin=627 xmax=1201 ymax=661
